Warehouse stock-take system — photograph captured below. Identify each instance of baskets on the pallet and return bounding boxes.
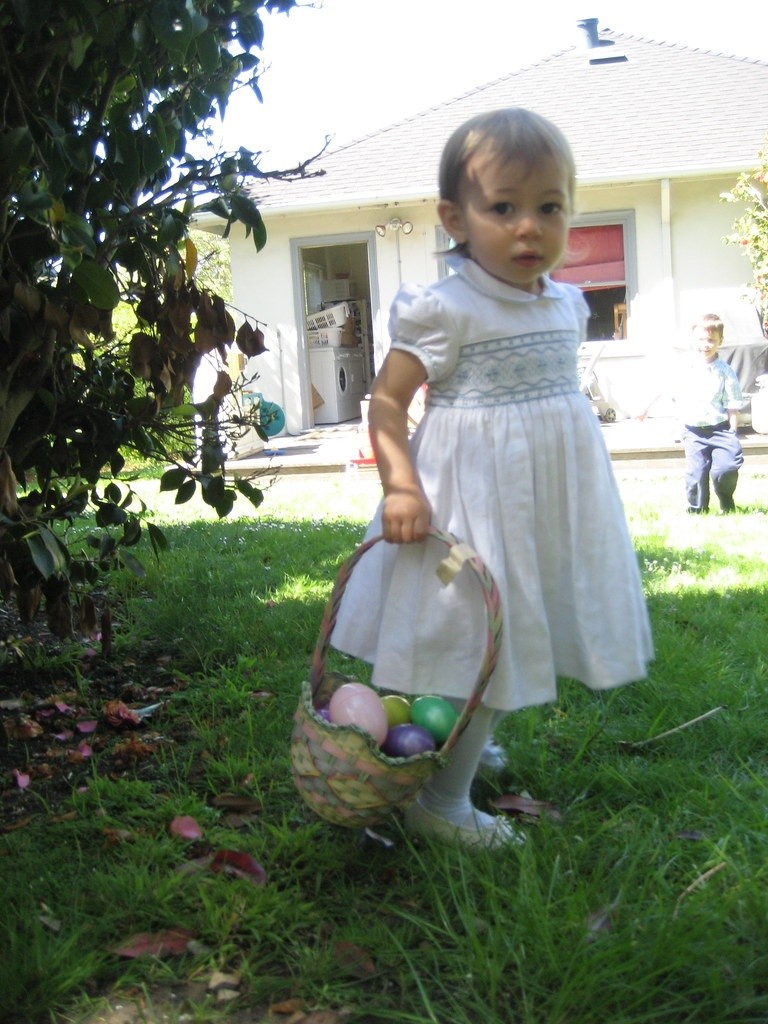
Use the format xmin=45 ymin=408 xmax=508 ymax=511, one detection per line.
xmin=304 ymin=327 xmax=345 ymax=348
xmin=305 ymin=306 xmax=349 ymax=329
xmin=290 ymin=522 xmax=501 ymax=830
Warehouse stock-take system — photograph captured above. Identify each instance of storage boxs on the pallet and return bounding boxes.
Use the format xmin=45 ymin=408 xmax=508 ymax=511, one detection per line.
xmin=307 ymin=328 xmax=345 ymax=348
xmin=305 ymin=299 xmax=349 ymax=329
xmin=340 ymin=317 xmax=356 ymax=346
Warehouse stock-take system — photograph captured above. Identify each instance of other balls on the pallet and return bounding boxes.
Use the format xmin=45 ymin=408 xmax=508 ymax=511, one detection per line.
xmin=315 ymin=681 xmax=461 ymax=762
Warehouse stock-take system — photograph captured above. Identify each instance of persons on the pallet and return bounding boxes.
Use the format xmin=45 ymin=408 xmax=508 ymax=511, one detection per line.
xmin=678 ymin=313 xmax=747 ymax=516
xmin=331 ymin=106 xmax=663 ymax=859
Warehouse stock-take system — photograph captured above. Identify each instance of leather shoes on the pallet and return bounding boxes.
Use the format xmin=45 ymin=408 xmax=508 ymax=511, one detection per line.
xmin=403 ymin=797 xmax=527 ymax=849
xmin=475 ymin=742 xmax=505 ymax=784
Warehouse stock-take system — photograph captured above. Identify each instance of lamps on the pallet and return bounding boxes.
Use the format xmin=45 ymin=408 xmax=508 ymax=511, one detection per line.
xmin=374 ymin=222 xmax=390 ymax=237
xmin=395 ymin=221 xmax=414 ymax=235
